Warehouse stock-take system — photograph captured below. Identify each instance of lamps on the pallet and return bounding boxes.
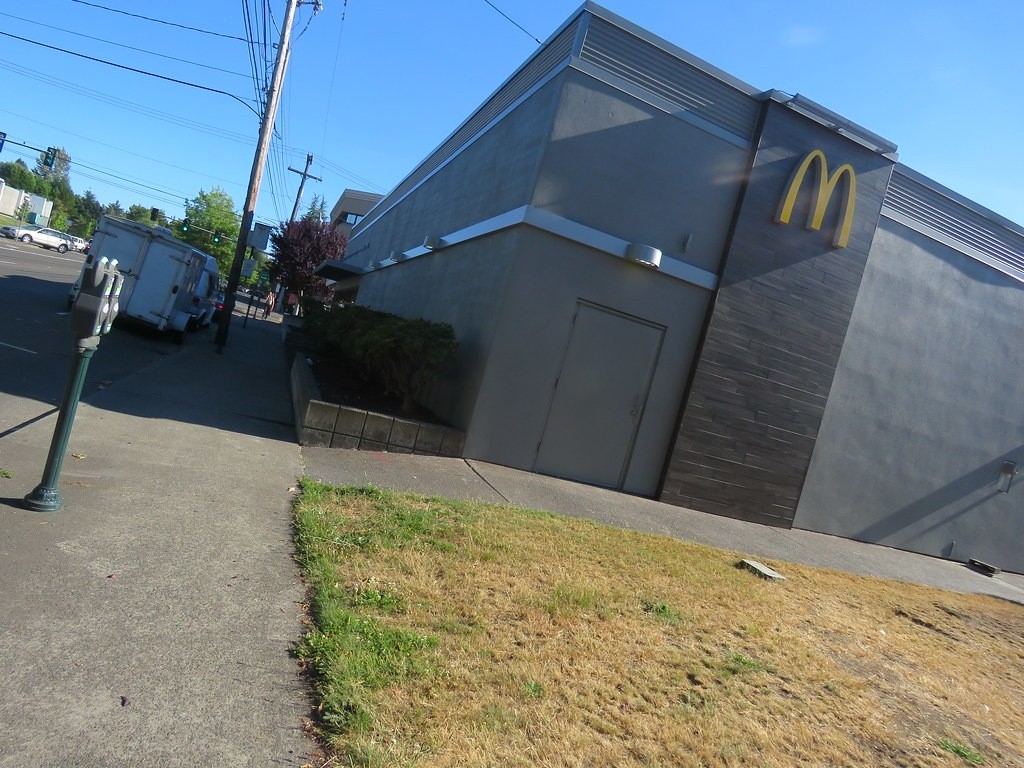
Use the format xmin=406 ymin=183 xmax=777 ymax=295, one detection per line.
xmin=368 ymin=259 xmax=379 ymax=271
xmin=625 ymin=242 xmax=662 ymax=270
xmin=423 ymin=234 xmax=441 ymax=251
xmin=390 ymin=249 xmax=403 ymax=262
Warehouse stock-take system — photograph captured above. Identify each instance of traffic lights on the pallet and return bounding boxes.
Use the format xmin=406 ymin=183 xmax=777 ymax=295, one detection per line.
xmin=182 ymin=218 xmax=191 ymax=231
xmin=151 ymin=207 xmax=158 ymax=221
xmin=214 ymin=229 xmax=222 ymax=244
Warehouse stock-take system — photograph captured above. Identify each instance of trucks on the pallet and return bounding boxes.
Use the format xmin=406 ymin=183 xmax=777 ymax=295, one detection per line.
xmin=68 ymin=213 xmax=221 ymax=341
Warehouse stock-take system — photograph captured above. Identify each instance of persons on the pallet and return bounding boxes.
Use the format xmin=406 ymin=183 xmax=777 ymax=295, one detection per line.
xmin=261 ymin=292 xmax=274 ymax=319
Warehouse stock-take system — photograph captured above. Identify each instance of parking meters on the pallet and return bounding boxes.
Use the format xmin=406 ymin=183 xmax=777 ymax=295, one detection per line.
xmin=23 ymin=255 xmax=125 ymax=511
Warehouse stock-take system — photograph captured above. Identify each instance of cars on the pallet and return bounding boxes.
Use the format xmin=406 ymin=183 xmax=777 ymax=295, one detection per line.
xmin=242 ymin=287 xmax=265 ymax=298
xmin=213 ymin=295 xmax=225 ymax=321
xmin=71 ymin=236 xmax=93 ymax=255
xmin=0 ymin=223 xmax=74 ymax=254
